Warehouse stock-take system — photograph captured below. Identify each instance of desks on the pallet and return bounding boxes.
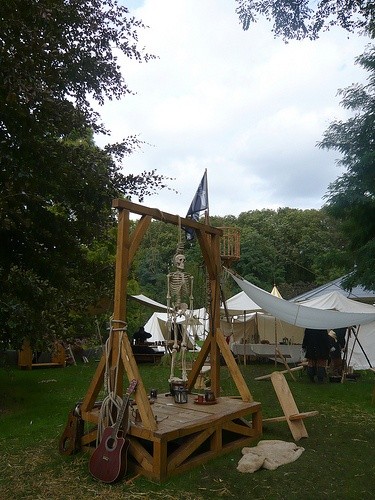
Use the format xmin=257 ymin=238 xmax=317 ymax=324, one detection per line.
xmin=230 ymin=342 xmax=303 ymax=366
xmin=132 ymin=343 xmax=172 ymax=359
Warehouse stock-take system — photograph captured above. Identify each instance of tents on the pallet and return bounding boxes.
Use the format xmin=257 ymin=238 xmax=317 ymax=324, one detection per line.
xmin=218 ymin=291 xmax=375 ymax=370
xmin=142 ymin=307 xmax=209 ymax=352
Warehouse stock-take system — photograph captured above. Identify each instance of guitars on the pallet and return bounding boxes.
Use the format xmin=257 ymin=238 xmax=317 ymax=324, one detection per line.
xmin=89 ymin=378 xmax=138 ymax=484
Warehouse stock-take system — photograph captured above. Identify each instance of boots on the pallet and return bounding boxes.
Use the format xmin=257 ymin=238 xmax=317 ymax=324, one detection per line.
xmin=307 ymin=365 xmax=326 ymax=384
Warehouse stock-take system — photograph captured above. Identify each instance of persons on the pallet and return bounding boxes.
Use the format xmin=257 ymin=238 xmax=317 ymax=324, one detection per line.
xmin=302 ymin=328 xmax=348 ymax=382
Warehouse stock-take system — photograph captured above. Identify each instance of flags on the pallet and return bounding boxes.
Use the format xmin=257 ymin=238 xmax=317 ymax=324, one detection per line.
xmin=180 ymin=169 xmax=208 ymax=242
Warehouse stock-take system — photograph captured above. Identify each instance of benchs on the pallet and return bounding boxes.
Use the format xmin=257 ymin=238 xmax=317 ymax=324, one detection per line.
xmin=132 ymin=353 xmax=164 ymax=364
